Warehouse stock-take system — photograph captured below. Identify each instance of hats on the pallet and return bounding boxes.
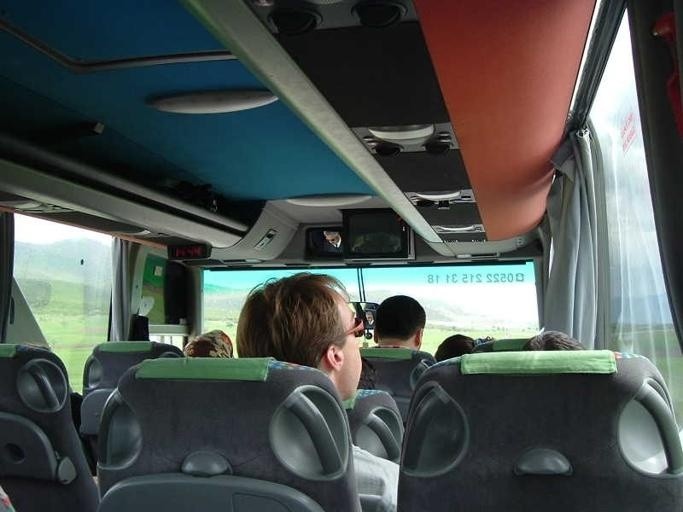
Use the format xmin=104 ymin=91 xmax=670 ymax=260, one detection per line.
xmin=184 ymin=329 xmax=233 ymax=357
xmin=324 ymin=231 xmax=339 ymax=240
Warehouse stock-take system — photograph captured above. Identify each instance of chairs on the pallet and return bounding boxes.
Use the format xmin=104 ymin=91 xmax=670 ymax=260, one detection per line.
xmin=0 ymin=324 xmax=682 ymax=512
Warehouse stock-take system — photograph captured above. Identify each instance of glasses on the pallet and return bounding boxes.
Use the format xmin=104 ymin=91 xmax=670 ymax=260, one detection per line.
xmin=325 ymin=317 xmax=367 ymax=354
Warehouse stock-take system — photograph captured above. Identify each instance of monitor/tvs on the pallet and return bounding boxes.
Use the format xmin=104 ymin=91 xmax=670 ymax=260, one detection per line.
xmin=342 ymin=209 xmax=409 ymax=260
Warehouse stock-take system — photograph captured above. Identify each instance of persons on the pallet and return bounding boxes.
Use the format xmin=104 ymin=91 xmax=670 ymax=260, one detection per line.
xmin=371 ymin=291 xmax=426 ymax=353
xmin=235 ymin=269 xmax=404 ymax=510
